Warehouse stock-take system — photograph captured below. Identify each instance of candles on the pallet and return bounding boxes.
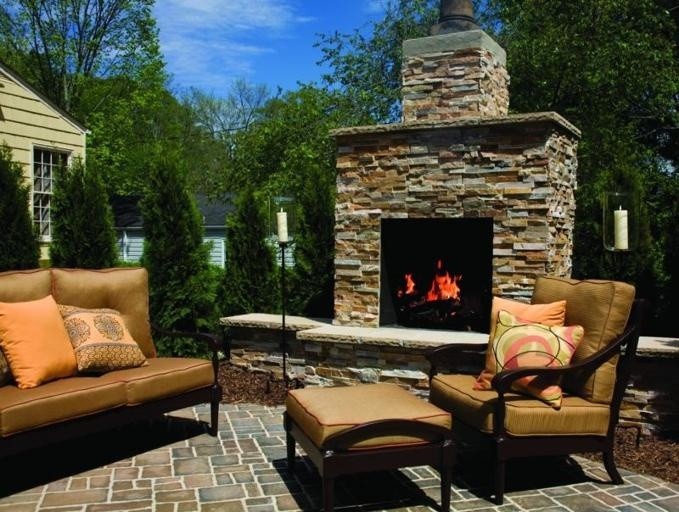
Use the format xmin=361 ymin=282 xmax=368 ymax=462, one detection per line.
xmin=613 ymin=204 xmax=629 ymax=250
xmin=276 ymin=207 xmax=289 ymax=242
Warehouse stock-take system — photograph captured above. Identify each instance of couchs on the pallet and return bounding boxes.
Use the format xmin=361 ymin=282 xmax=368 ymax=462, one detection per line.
xmin=0 ymin=265 xmax=224 ymax=459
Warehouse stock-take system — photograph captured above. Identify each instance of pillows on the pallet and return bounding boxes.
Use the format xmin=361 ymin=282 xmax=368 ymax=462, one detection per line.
xmin=0 ymin=295 xmax=150 ymax=389
xmin=473 ymin=295 xmax=585 ymax=411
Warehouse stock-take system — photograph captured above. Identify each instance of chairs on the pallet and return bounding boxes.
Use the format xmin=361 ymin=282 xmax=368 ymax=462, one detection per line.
xmin=430 ymin=274 xmax=645 ymax=504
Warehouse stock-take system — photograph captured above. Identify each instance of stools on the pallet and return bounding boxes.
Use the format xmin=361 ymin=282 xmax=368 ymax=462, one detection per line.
xmin=283 ymin=381 xmax=453 ymax=511
